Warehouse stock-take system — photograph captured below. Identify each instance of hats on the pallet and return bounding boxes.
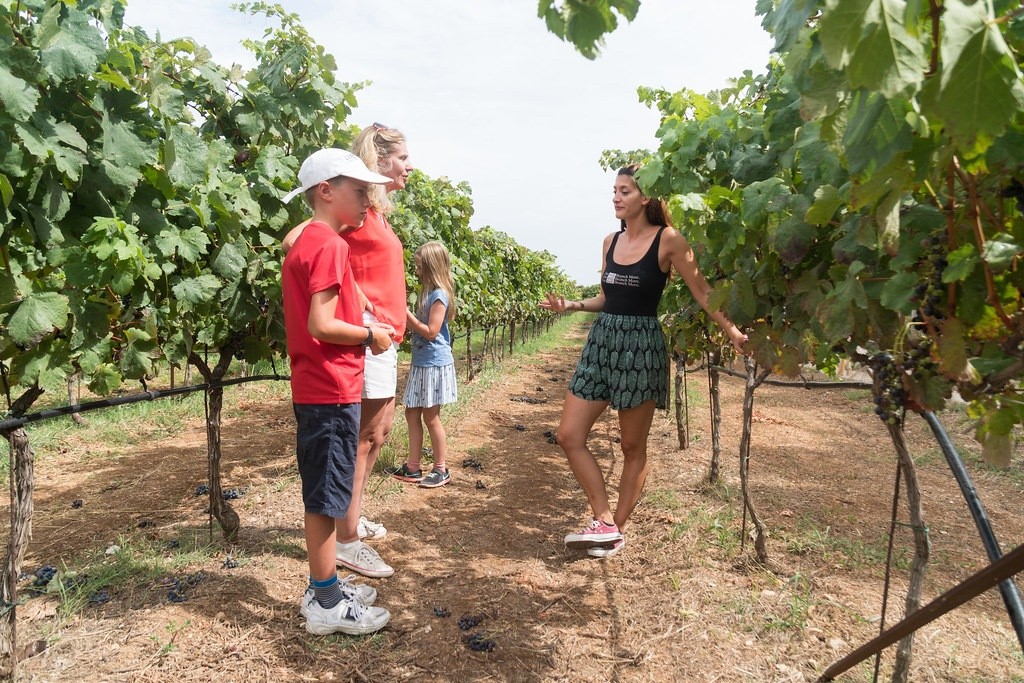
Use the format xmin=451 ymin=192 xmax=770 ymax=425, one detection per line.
xmin=281 ymin=148 xmax=395 ymax=205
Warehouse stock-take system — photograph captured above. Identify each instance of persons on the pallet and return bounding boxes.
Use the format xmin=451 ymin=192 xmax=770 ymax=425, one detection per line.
xmin=281 ymin=146 xmax=399 ymax=637
xmin=283 ymin=122 xmax=413 ymax=579
xmin=536 ymin=162 xmax=754 ymax=560
xmin=382 ymin=242 xmax=458 ymax=489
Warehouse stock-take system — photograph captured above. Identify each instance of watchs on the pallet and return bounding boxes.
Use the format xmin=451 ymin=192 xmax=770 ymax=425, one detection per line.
xmin=357 ymin=326 xmax=374 ymax=348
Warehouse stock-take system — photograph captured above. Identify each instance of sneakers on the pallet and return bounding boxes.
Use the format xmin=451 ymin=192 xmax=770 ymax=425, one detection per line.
xmin=335 ymin=516 xmax=394 ymax=578
xmin=300 ymin=578 xmax=390 ymax=636
xmin=384 ymin=463 xmax=423 ymax=482
xmin=564 ymin=517 xmax=625 ymax=557
xmin=419 ymin=468 xmax=452 ymax=488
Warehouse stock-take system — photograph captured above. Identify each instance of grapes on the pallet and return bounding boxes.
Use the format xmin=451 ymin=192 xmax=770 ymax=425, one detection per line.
xmin=30 ymin=565 xmax=109 ymax=604
xmin=461 ymin=459 xmax=484 ymax=471
xmin=912 ymin=237 xmax=950 ymax=337
xmin=193 ymin=485 xmax=247 ymax=500
xmin=169 ymin=568 xmax=204 ymax=603
xmin=871 ymin=349 xmax=916 ymax=424
xmin=434 ymin=607 xmax=496 ymax=652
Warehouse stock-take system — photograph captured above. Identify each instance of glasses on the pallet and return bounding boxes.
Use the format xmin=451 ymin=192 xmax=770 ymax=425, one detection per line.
xmin=373 ymin=122 xmax=389 ymax=142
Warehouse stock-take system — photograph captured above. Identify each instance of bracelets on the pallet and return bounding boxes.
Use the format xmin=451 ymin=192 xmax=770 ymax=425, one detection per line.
xmin=578 ymin=302 xmax=585 ymax=311
